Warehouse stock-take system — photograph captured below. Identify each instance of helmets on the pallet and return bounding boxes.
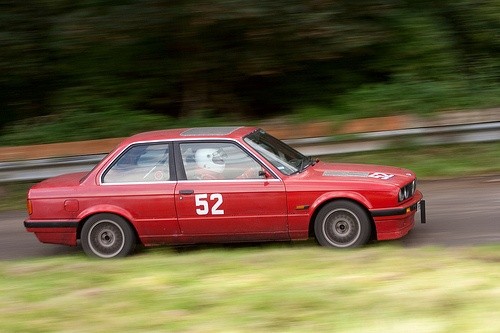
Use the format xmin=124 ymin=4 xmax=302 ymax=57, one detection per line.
xmin=195 ymin=148 xmax=228 ymax=173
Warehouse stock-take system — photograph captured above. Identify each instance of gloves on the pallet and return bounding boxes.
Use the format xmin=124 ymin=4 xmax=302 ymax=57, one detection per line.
xmin=242 ymin=166 xmax=258 ymax=177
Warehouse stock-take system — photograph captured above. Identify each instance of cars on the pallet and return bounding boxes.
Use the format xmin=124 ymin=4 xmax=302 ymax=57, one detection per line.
xmin=22 ymin=124 xmax=427 ymax=259
